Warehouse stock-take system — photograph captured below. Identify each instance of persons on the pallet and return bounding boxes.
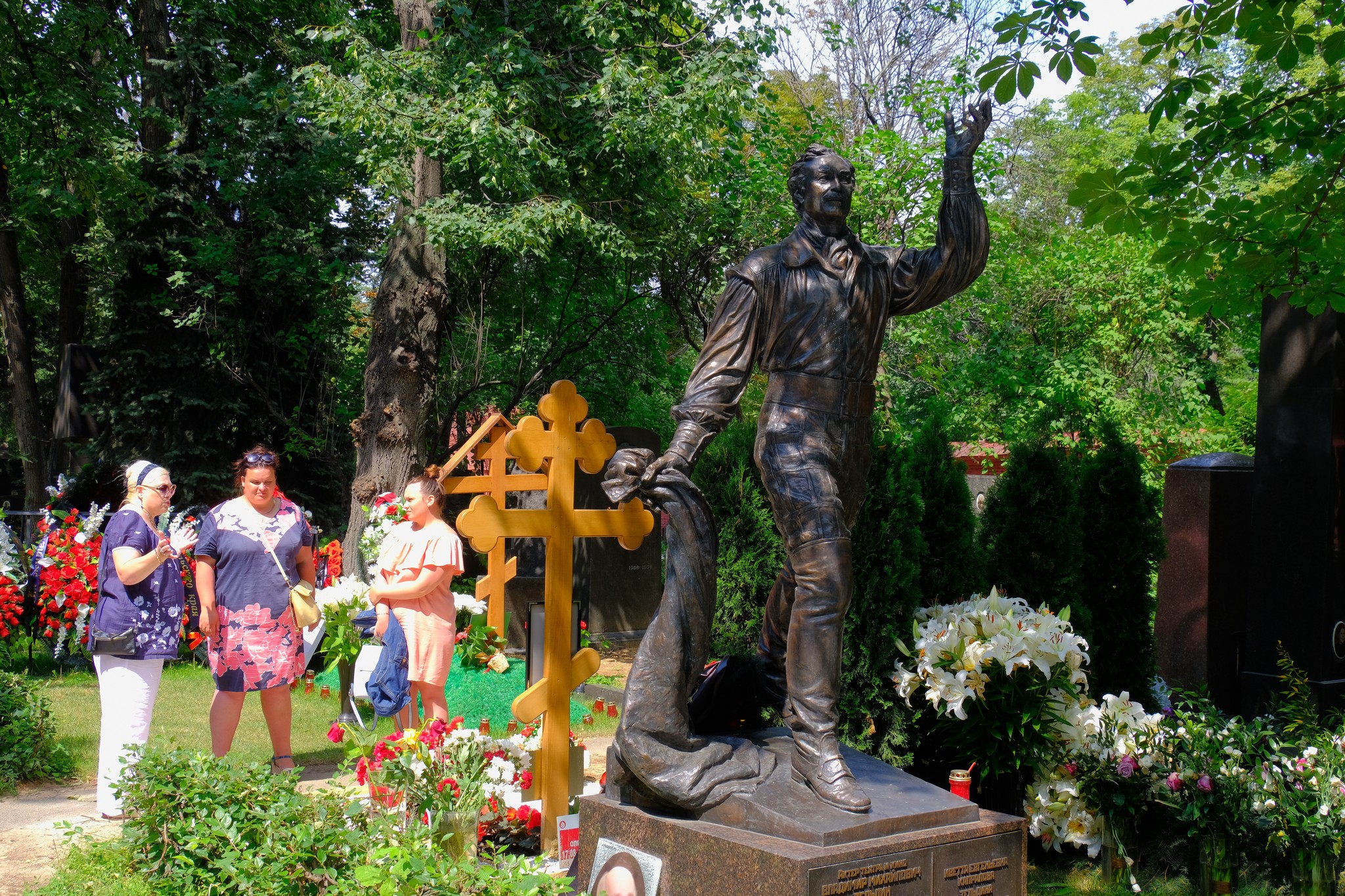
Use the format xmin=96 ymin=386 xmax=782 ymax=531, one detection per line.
xmin=90 ymin=459 xmax=201 ymax=820
xmin=192 ymin=447 xmax=317 ymax=787
xmin=640 ymin=100 xmax=992 ymax=812
xmin=368 ymin=464 xmax=464 ymax=732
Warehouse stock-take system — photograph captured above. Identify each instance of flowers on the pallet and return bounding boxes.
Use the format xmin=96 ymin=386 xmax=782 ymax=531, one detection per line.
xmin=313 ymin=491 xmax=510 ymax=674
xmin=327 ymin=716 xmax=602 ymax=843
xmin=0 ymin=474 xmax=209 ymax=666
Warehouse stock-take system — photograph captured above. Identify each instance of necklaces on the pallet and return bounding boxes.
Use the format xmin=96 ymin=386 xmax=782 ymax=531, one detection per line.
xmin=243 ymin=496 xmax=276 ymax=515
xmin=131 ymin=503 xmax=159 ymax=532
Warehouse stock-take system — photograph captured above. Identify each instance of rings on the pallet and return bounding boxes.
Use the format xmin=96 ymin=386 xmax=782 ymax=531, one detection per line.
xmin=202 ymin=629 xmax=206 ymax=631
xmin=188 ymin=539 xmax=191 ymax=542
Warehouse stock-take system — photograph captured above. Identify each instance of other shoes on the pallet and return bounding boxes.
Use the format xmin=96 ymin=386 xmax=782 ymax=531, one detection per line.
xmin=101 ymin=810 xmax=145 ymax=819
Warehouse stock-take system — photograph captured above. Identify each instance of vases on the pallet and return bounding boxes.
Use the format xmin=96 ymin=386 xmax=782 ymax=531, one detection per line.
xmin=335 ymin=660 xmax=362 ymax=725
xmin=426 ymin=803 xmax=484 ymax=867
xmin=366 ymin=767 xmax=408 ymax=848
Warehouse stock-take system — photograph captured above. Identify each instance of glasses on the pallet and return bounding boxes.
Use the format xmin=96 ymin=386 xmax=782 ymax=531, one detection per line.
xmin=244 ymin=454 xmax=275 ymax=463
xmin=138 ymin=484 xmax=176 ymax=496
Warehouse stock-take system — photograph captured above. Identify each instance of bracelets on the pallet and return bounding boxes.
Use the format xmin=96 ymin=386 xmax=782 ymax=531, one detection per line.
xmin=377 ymin=612 xmax=389 ymax=615
xmin=315 ymin=602 xmax=317 ymax=604
xmin=155 ymin=548 xmax=164 ymax=565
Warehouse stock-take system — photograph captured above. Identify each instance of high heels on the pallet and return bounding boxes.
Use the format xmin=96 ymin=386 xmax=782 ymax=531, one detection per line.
xmin=271 ymin=755 xmax=298 ymax=778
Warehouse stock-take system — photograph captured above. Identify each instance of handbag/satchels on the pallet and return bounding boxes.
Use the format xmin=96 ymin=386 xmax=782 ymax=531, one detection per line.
xmin=348 ymin=635 xmax=412 ymax=733
xmin=289 ymin=579 xmax=322 ymax=629
